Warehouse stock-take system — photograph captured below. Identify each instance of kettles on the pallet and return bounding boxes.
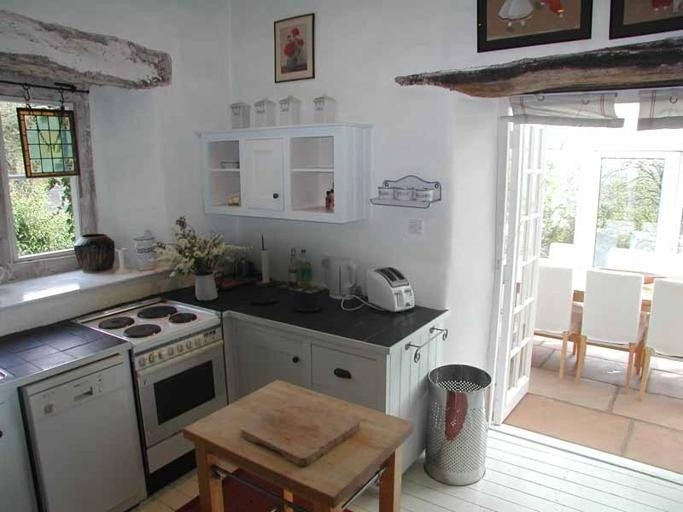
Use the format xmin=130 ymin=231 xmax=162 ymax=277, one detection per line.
xmin=326 ymin=256 xmax=356 ymax=301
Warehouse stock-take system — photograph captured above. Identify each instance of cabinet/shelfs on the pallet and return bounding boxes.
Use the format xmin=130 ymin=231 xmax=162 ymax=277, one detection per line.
xmin=203 ymin=133 xmax=244 ymax=216
xmin=288 ymin=127 xmax=372 ymax=224
xmin=246 ymin=136 xmax=286 ymax=217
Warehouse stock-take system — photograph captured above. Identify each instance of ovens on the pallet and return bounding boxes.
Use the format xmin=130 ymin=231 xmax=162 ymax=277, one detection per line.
xmin=131 ymin=324 xmax=230 ymax=496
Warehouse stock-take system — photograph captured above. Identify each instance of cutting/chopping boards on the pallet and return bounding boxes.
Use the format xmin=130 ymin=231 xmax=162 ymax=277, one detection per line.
xmin=241 ymin=401 xmax=362 ymax=466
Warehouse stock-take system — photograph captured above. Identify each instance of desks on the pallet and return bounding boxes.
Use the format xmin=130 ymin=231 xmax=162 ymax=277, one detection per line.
xmin=184 ymin=379 xmax=416 ymax=512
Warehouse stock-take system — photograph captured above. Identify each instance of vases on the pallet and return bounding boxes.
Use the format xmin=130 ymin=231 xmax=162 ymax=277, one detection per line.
xmin=194 ymin=274 xmax=219 ymax=301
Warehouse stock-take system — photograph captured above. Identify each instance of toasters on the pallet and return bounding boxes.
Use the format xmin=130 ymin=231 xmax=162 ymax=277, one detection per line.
xmin=365 ymin=265 xmax=417 ymax=314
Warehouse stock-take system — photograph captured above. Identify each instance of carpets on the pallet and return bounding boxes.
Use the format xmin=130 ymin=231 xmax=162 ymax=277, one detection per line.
xmin=176 ymin=468 xmax=352 ymax=512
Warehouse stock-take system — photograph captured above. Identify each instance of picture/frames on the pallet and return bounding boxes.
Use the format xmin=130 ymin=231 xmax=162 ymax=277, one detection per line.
xmin=477 ymin=0 xmax=592 ymax=52
xmin=609 ymin=1 xmax=683 ymax=40
xmin=272 ymin=13 xmax=318 ymax=83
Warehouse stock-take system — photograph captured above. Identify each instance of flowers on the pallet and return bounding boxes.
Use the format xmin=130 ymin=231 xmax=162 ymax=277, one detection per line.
xmin=172 ymin=215 xmax=235 ymax=275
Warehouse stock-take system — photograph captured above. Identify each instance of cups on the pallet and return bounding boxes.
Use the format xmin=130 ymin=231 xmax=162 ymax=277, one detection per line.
xmin=377 ymin=185 xmax=435 ymax=202
xmin=131 ymin=235 xmax=159 ymax=272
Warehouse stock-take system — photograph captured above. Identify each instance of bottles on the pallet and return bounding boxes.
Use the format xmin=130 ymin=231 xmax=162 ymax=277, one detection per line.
xmin=229 ymin=95 xmax=338 ymax=130
xmin=285 ymin=244 xmax=312 ymax=291
xmin=73 ymin=233 xmax=115 ymax=273
xmin=325 ymin=189 xmax=334 ymax=210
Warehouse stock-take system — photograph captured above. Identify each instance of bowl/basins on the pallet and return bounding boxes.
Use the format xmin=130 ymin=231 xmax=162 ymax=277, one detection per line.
xmin=221 ymin=161 xmax=239 ymax=169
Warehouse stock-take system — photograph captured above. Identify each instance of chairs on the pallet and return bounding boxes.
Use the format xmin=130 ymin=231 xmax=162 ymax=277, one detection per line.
xmin=576 ymin=268 xmax=647 ymax=393
xmin=532 ymin=264 xmax=574 ymax=377
xmin=640 ymin=277 xmax=683 ymax=396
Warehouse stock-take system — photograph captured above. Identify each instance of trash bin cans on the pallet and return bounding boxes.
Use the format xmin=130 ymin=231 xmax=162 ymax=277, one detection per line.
xmin=424 ymin=364 xmax=492 ymax=486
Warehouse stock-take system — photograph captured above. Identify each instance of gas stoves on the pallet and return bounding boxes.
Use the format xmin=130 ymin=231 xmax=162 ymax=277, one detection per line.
xmin=70 ymin=296 xmax=222 ymax=370
